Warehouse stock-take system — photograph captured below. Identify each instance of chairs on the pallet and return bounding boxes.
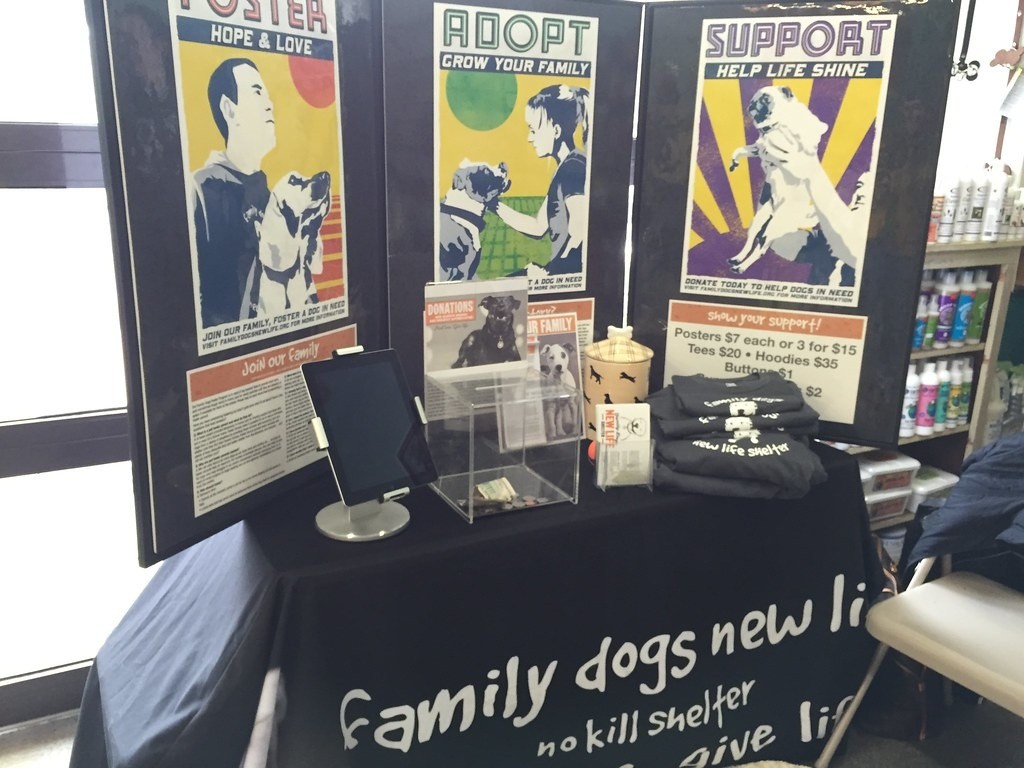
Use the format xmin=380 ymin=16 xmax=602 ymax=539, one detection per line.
xmin=815 ymin=432 xmax=1024 ymax=768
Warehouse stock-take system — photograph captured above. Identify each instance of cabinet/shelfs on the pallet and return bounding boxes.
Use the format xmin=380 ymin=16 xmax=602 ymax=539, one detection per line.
xmin=869 ymin=233 xmax=1024 ymax=533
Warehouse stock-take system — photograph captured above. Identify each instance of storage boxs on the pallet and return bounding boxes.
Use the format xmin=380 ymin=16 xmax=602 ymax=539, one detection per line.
xmin=424 ymin=366 xmax=583 ymax=523
xmin=859 ymin=469 xmax=874 ymax=494
xmin=867 ymin=488 xmax=912 ymax=522
xmin=592 ymin=438 xmax=656 ymax=493
xmin=905 ymin=465 xmax=960 ymax=513
xmin=858 ymin=450 xmax=921 ymax=492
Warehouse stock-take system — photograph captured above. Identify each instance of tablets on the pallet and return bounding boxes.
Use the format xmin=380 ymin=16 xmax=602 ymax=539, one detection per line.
xmin=299 ymin=348 xmax=438 ymax=507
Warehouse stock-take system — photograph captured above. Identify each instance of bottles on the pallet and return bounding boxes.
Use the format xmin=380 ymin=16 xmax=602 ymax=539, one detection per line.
xmin=897 ymin=159 xmax=1024 ymax=439
xmin=584 ymin=325 xmax=653 ymax=442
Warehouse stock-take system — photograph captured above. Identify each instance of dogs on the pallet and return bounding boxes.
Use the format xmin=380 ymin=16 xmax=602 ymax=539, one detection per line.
xmin=539 ymin=342 xmax=578 ymax=437
xmin=451 ymin=294 xmax=521 ymax=370
